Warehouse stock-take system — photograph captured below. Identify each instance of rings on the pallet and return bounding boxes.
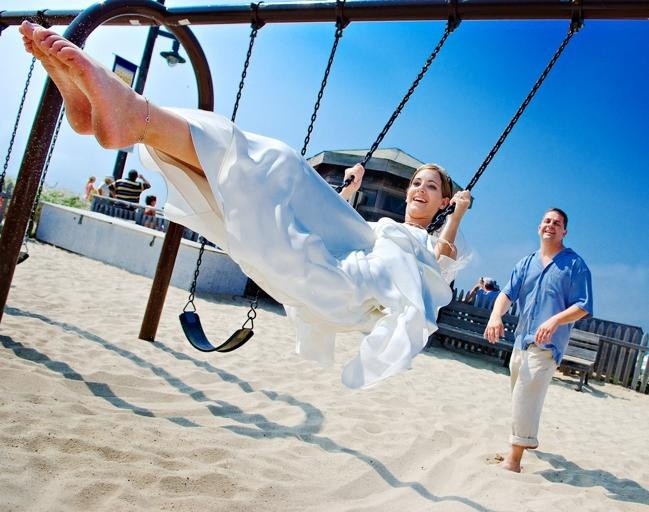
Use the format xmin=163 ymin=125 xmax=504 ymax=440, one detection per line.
xmin=459 ymin=194 xmax=464 ymax=199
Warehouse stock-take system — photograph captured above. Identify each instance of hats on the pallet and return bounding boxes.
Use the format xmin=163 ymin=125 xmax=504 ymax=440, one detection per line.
xmin=484 ymin=278 xmax=493 ymax=285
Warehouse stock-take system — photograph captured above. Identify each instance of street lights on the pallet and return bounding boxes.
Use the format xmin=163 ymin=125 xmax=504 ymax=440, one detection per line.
xmin=106 ymin=0 xmax=187 ymax=197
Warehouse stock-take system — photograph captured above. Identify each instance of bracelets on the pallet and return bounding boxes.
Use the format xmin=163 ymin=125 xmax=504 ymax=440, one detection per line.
xmin=434 ymin=237 xmax=457 ymax=255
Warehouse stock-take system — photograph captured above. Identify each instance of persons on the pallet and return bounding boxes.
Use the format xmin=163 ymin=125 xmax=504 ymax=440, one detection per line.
xmin=107 ymin=168 xmax=152 ymax=220
xmin=482 ymin=207 xmax=593 ymax=474
xmin=137 ymin=195 xmax=158 ymax=230
xmin=82 ymin=175 xmax=97 ymax=203
xmin=96 ymin=174 xmax=115 ymax=197
xmin=463 ymin=277 xmax=502 ymax=325
xmin=17 ymin=20 xmax=472 ymax=335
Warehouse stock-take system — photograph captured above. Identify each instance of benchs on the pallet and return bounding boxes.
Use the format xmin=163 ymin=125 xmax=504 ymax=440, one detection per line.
xmin=561 ymin=327 xmax=601 ymax=391
xmin=427 ymin=301 xmax=519 ymax=368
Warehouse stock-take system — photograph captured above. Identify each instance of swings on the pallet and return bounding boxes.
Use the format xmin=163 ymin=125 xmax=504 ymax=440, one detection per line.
xmin=179 ymin=28 xmax=343 ymax=352
xmin=0 ymin=25 xmax=86 ymax=265
